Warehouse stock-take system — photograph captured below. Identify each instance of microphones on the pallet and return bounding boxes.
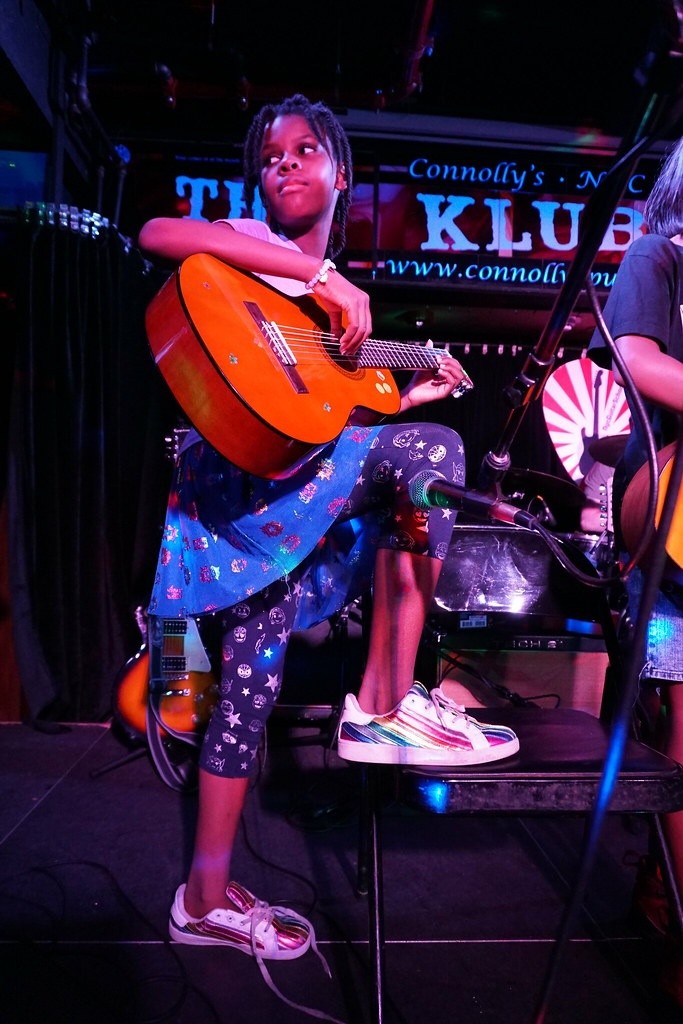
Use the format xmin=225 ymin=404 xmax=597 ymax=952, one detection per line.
xmin=408 ymin=470 xmax=534 ymax=529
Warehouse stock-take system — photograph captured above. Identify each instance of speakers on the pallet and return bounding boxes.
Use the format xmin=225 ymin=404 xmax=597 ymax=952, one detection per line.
xmin=434 ymin=632 xmax=613 ymax=723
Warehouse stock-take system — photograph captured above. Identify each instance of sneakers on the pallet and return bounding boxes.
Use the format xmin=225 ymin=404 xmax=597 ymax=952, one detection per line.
xmin=335 ymin=680 xmax=522 ymax=766
xmin=167 ymin=877 xmax=349 ymax=1023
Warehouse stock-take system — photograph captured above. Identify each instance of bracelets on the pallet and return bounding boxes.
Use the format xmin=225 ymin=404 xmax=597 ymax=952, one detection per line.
xmin=305 ymin=259 xmax=337 ymax=290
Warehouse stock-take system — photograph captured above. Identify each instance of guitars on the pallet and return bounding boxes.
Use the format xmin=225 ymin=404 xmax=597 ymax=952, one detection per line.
xmin=620 ymin=439 xmax=683 ymax=574
xmin=143 ymin=251 xmax=475 ymax=481
xmin=113 ymin=431 xmax=220 ymax=743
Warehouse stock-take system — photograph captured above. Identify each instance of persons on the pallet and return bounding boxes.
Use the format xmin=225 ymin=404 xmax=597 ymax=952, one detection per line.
xmin=585 ymin=138 xmax=683 ymax=934
xmin=140 ymin=93 xmax=522 ymax=960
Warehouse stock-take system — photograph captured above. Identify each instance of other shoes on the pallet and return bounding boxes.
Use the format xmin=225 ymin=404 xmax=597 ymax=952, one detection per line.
xmin=622 ymin=848 xmax=683 ymax=941
